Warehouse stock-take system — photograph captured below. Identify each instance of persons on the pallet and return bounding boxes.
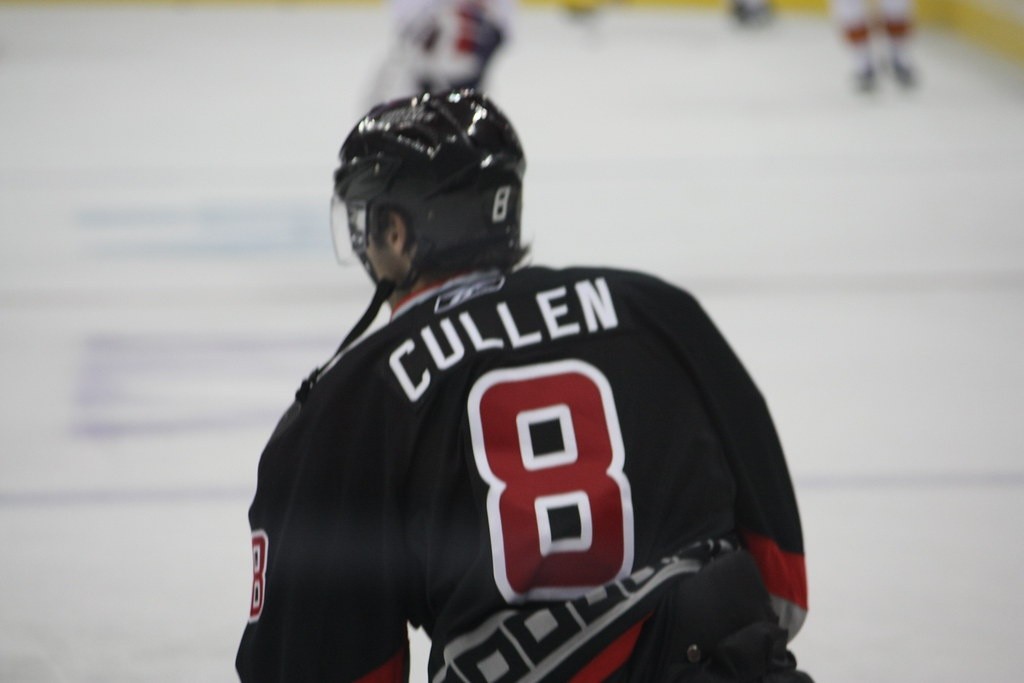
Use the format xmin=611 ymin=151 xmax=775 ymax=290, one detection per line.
xmin=393 ymin=0 xmax=925 ymax=97
xmin=236 ymin=91 xmax=815 ymax=683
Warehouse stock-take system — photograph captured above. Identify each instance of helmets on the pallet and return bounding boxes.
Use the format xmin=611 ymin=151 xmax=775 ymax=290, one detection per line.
xmin=334 ymin=88 xmax=525 ymax=264
